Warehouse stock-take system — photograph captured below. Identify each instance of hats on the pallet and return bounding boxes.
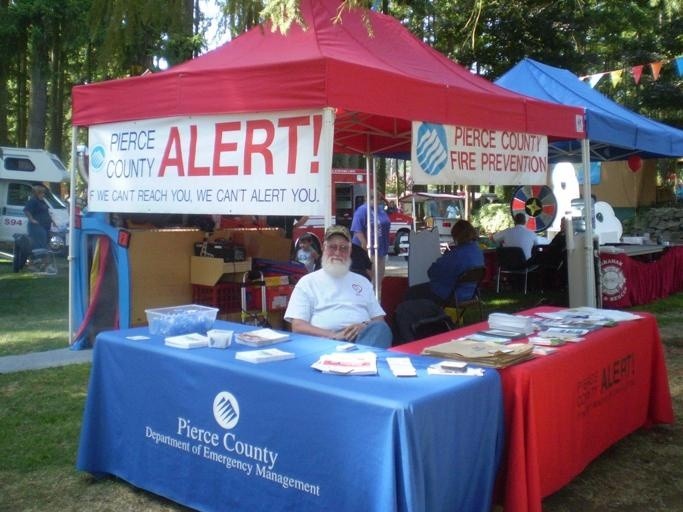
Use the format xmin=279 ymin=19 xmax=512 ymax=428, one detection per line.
xmin=324 ymin=225 xmax=351 ymax=242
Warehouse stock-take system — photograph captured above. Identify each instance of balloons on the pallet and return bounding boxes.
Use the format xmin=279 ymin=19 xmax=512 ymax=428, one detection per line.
xmin=628 ymin=155 xmax=642 ymax=172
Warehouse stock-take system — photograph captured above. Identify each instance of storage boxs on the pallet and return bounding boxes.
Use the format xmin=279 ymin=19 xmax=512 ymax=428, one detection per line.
xmin=144 ymin=302 xmax=221 ymax=335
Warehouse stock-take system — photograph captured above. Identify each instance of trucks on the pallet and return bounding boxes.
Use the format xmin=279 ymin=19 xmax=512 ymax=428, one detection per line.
xmin=220 ymin=168 xmax=412 ymax=257
xmin=0 ymin=144 xmax=69 ymax=254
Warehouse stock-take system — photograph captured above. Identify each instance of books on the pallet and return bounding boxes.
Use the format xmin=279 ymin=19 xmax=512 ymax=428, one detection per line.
xmin=318 ymin=352 xmax=377 ymax=375
xmin=419 ymin=304 xmax=642 ymax=379
xmin=385 ymin=357 xmax=416 ymax=377
xmin=233 ymin=328 xmax=290 ymax=346
xmin=335 ymin=344 xmax=355 ymax=352
xmin=164 ymin=333 xmax=208 ymax=349
xmin=234 ymin=348 xmax=294 ymax=364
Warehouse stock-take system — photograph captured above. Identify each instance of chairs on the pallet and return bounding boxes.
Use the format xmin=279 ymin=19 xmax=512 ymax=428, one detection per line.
xmin=12 ymin=235 xmax=58 ymax=274
xmin=494 ymin=249 xmax=540 ymax=296
xmin=443 ymin=271 xmax=486 ymax=325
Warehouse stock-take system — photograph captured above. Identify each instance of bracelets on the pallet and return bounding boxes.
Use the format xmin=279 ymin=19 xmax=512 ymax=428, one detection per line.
xmin=362 ymin=320 xmax=369 ymax=327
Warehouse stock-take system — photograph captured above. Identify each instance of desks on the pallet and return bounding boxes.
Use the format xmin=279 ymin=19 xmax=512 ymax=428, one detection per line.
xmin=390 ymin=305 xmax=674 ymax=506
xmin=600 ymin=247 xmax=674 ymax=302
xmin=80 ymin=321 xmax=505 ymax=503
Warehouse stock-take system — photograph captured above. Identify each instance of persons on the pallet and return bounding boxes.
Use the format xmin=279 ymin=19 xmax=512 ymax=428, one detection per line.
xmin=349 ymin=189 xmax=390 ymax=304
xmin=489 ymin=212 xmax=550 ymax=271
xmin=444 ymin=200 xmax=459 ymax=219
xmin=548 ymin=216 xmax=567 ymax=269
xmin=282 ymin=223 xmax=392 ymax=349
xmin=281 ymin=216 xmax=311 ymax=261
xmin=295 ymin=234 xmax=319 ymax=273
xmin=20 ymin=185 xmax=51 ymax=270
xmin=406 ymin=219 xmax=484 ymax=301
xmin=386 ymin=200 xmax=398 ymax=215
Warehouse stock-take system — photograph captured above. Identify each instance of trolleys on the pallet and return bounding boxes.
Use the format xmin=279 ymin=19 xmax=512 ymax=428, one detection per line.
xmin=237 ymin=269 xmax=268 ymax=326
